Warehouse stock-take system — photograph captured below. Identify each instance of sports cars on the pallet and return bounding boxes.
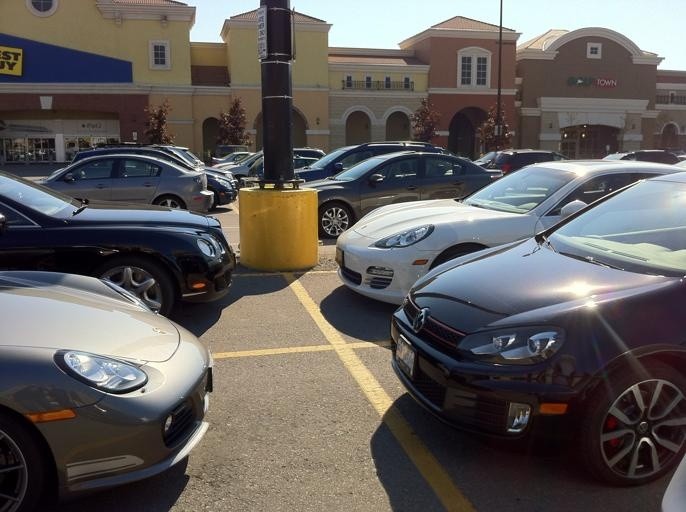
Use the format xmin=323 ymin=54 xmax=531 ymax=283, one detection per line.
xmin=1 ymin=269 xmax=215 ymax=512
xmin=335 ymin=160 xmax=686 ymax=313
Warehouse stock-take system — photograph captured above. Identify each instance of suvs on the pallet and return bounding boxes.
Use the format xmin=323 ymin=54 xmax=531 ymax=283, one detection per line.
xmin=390 ymin=173 xmax=684 ymax=487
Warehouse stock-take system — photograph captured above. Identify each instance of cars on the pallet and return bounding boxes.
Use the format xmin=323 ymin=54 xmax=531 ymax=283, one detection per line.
xmin=1 ymin=169 xmax=238 ymax=325
xmin=11 ymin=150 xmax=31 ymax=161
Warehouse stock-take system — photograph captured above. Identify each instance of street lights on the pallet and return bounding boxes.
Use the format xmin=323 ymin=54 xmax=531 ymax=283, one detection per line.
xmin=490 ymin=115 xmax=503 ymax=147
